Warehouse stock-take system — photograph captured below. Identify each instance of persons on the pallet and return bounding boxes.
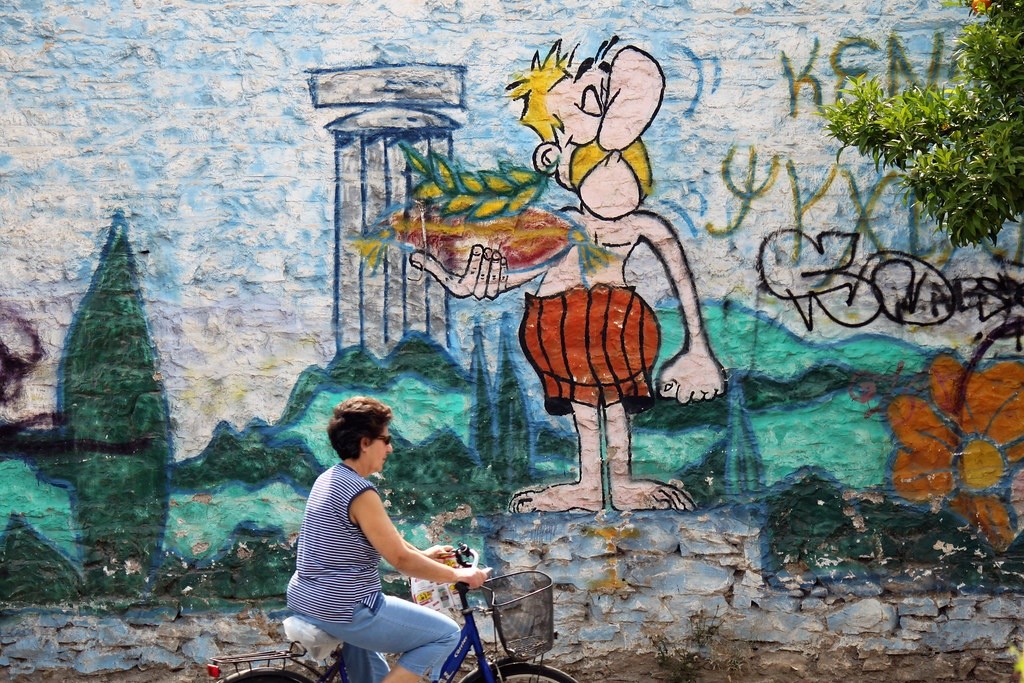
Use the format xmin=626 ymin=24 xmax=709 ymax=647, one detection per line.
xmin=287 ymin=396 xmax=485 ymax=683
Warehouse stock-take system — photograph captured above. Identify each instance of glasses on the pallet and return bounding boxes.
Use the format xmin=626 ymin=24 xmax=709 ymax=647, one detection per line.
xmin=366 ymin=435 xmax=392 ymax=444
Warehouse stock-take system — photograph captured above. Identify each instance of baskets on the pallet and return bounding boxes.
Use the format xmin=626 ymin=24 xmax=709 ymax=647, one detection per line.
xmin=480 ymin=570 xmax=553 ymax=662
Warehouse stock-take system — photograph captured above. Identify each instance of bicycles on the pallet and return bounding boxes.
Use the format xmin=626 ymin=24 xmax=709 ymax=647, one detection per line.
xmin=207 ymin=542 xmax=580 ymax=683
